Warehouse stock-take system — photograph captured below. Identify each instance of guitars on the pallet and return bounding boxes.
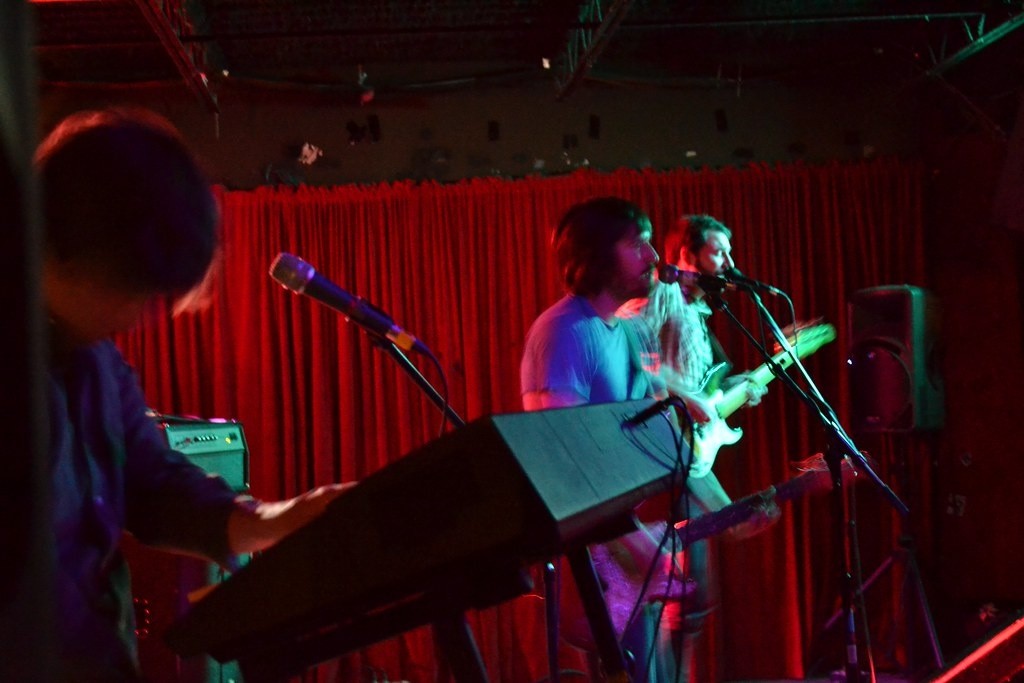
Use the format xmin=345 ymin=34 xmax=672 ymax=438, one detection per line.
xmin=567 ymin=449 xmax=875 ymax=653
xmin=685 ymin=318 xmax=834 ymax=480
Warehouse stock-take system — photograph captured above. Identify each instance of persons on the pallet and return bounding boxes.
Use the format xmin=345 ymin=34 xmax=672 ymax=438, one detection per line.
xmin=521 ymin=197 xmax=778 ymax=683
xmin=638 ymin=213 xmax=770 ymax=637
xmin=32 ymin=105 xmax=360 ymax=683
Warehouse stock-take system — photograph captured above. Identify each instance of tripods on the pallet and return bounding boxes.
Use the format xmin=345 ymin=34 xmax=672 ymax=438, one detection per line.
xmin=820 ymin=441 xmax=950 ymax=669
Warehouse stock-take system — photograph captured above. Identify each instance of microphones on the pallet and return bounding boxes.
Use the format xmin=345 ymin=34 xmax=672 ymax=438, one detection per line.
xmin=658 ymin=264 xmax=736 ymax=295
xmin=728 ymin=266 xmax=772 ymax=293
xmin=272 ymin=253 xmax=428 ymax=360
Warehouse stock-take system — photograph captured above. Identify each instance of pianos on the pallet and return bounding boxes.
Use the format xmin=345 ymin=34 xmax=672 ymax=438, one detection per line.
xmin=163 ymin=395 xmax=697 ymax=664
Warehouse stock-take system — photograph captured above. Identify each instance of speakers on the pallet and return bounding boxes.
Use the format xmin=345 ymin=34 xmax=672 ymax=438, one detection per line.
xmin=848 ymin=285 xmax=947 ymax=436
